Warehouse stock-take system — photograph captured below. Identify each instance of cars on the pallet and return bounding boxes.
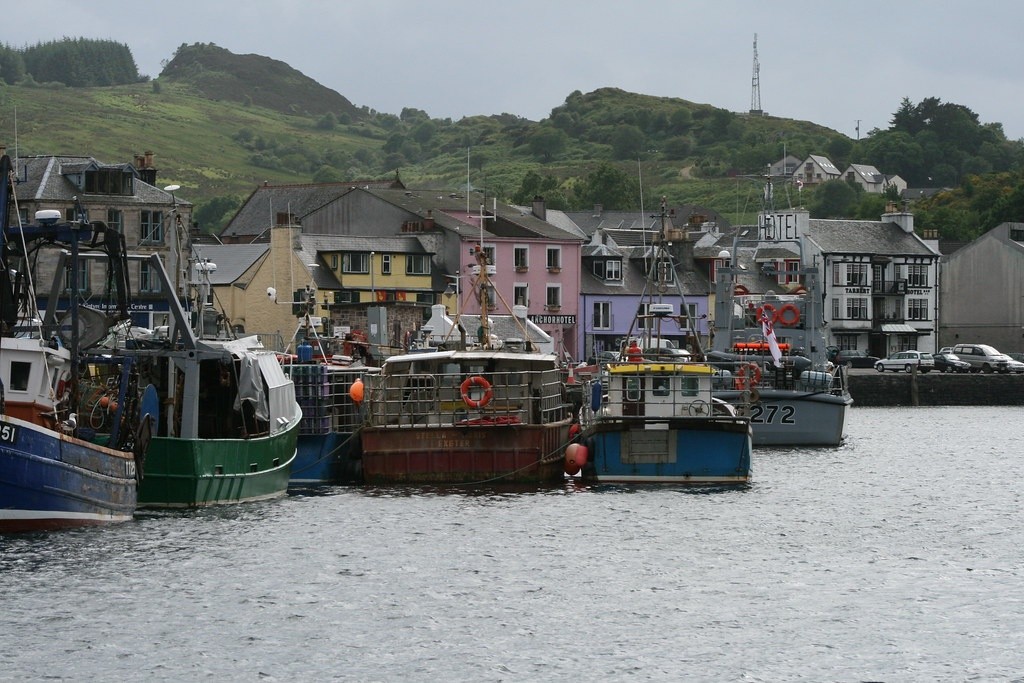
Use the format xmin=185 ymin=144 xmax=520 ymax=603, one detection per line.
xmin=932 ymin=343 xmax=1024 ymax=374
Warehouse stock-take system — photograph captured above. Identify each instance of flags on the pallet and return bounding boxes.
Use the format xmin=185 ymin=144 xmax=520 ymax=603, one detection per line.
xmin=762 ymin=310 xmax=782 ymax=360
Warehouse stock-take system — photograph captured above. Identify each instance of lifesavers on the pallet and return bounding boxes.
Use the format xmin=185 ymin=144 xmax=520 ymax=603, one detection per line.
xmin=739 ymin=365 xmax=761 ymax=386
xmin=778 ymin=304 xmax=800 ymax=325
xmin=757 ymin=304 xmax=777 ymax=325
xmin=460 ymin=377 xmax=492 ymax=407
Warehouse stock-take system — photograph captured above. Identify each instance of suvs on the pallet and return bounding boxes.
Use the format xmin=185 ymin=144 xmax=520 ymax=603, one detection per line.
xmin=832 ymin=349 xmax=881 ymax=369
xmin=873 ymin=349 xmax=936 ymax=373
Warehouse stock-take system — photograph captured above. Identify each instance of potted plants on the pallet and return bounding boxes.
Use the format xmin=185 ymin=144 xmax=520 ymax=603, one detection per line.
xmin=550 ymin=266 xmax=562 ymax=271
xmin=517 ymin=266 xmax=528 ymax=273
xmin=879 ymin=180 xmax=904 ymax=212
xmin=487 ymin=304 xmax=495 ymax=310
xmin=549 ymin=304 xmax=561 ymax=310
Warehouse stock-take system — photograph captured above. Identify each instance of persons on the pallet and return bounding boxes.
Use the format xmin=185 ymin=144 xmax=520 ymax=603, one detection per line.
xmin=343 ymin=334 xmax=353 ymax=355
xmin=621 ymin=340 xmax=642 ymax=361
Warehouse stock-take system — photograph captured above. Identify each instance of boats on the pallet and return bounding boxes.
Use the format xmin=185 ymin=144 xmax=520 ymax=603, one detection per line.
xmin=0 ymin=101 xmax=855 ymax=536
